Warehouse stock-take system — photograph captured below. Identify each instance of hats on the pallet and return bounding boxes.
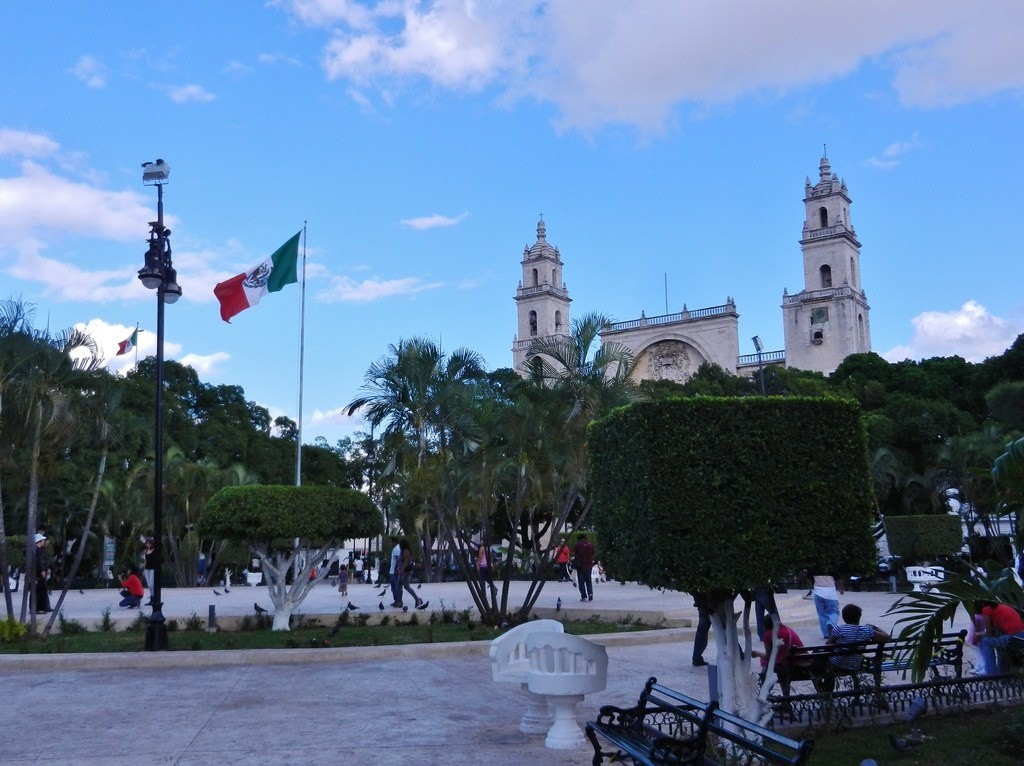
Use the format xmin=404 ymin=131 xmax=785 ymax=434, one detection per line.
xmin=145 ymin=537 xmax=153 ymax=542
xmin=561 ymin=539 xmax=565 ymax=542
xmin=36 ymin=534 xmax=47 ymax=542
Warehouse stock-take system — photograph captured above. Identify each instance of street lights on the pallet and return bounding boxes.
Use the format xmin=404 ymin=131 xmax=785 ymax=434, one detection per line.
xmin=137 ymin=158 xmax=183 ymax=652
xmin=750 ymin=335 xmax=766 ymax=395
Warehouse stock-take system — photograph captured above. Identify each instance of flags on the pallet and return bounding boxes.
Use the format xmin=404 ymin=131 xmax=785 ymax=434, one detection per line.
xmin=117 ymin=328 xmax=137 ymax=355
xmin=214 ymin=231 xmax=302 ymax=324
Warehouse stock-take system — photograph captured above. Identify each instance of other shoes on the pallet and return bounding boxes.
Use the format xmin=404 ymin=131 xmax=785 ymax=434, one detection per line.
xmin=589 ymin=594 xmax=593 ymax=601
xmin=394 ymin=602 xmax=403 ymax=606
xmin=976 ymin=671 xmax=988 ymax=675
xmin=580 ymin=598 xmax=587 ymax=601
xmin=827 ymin=624 xmax=833 ymax=635
xmin=415 ymin=598 xmax=423 ymax=607
xmin=391 ymin=601 xmax=396 ymax=606
xmin=824 ymin=636 xmax=829 ymax=639
xmin=970 ymin=669 xmax=981 ymax=673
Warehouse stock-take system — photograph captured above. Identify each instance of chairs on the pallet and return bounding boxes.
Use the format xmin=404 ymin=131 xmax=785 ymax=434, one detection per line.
xmin=526 ymin=631 xmax=609 ymax=750
xmin=490 ymin=619 xmax=564 ymax=734
xmin=906 ymin=566 xmax=944 ymax=593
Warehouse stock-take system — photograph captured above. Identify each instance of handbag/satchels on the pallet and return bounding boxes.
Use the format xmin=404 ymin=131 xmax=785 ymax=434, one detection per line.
xmin=570 ymin=570 xmax=577 ymax=580
xmin=761 ymin=657 xmax=782 ymax=667
xmin=810 ymin=651 xmax=828 ymax=674
xmin=331 ymin=581 xmax=336 ymax=586
xmin=406 ymin=559 xmax=415 ymax=572
xmin=571 ymin=559 xmax=577 ymax=570
xmin=599 ymin=570 xmax=604 ymax=574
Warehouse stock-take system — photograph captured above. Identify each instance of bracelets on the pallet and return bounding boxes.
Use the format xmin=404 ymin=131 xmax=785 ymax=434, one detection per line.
xmin=873 ymin=625 xmax=878 ymax=629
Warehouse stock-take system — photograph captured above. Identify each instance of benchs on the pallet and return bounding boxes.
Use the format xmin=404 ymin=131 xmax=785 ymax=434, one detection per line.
xmin=586 ymin=676 xmax=814 ymax=766
xmin=759 ymin=630 xmax=968 ymax=709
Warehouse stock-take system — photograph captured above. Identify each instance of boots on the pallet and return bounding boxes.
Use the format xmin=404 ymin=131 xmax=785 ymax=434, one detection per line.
xmin=145 ymin=596 xmax=153 ymax=605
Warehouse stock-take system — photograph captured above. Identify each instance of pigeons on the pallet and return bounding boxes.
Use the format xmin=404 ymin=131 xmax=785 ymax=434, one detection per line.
xmin=348 ymin=601 xmax=360 ymax=612
xmin=419 ymin=601 xmax=429 ymax=611
xmin=213 ymin=589 xmax=222 ymax=596
xmin=557 ymin=596 xmax=562 ymax=611
xmin=224 ymin=587 xmax=231 ymax=594
xmin=373 ymin=580 xmax=390 ymax=589
xmin=253 ymin=602 xmax=268 ymax=615
xmin=403 ymin=605 xmax=408 ymax=612
xmin=416 ymin=583 xmax=421 ymax=590
xmin=379 ymin=601 xmax=385 ymax=612
xmin=573 ymin=577 xmax=606 ymax=587
xmin=377 ymin=588 xmax=386 ymax=597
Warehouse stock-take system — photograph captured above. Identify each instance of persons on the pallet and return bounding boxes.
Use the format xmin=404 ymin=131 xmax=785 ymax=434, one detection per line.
xmin=29 ymin=534 xmax=52 ymax=613
xmin=118 ymin=570 xmax=144 ymax=608
xmin=337 ymin=558 xmax=364 ymax=596
xmin=755 ymin=595 xmax=782 ymax=641
xmin=692 ymin=602 xmax=745 ymax=666
xmin=967 ymin=598 xmax=1024 ymax=676
xmin=389 ymin=537 xmax=423 ymax=608
xmin=751 ymin=616 xmax=823 ymax=711
xmin=556 ymin=536 xmax=607 ymax=601
xmin=143 ymin=539 xmax=154 ymax=605
xmin=308 ymin=565 xmax=324 ymax=582
xmin=478 ymin=541 xmax=498 ymax=594
xmin=197 ymin=573 xmax=206 ymax=586
xmin=811 ymin=576 xmax=841 ymax=637
xmin=825 ymin=605 xmax=891 ymax=692
